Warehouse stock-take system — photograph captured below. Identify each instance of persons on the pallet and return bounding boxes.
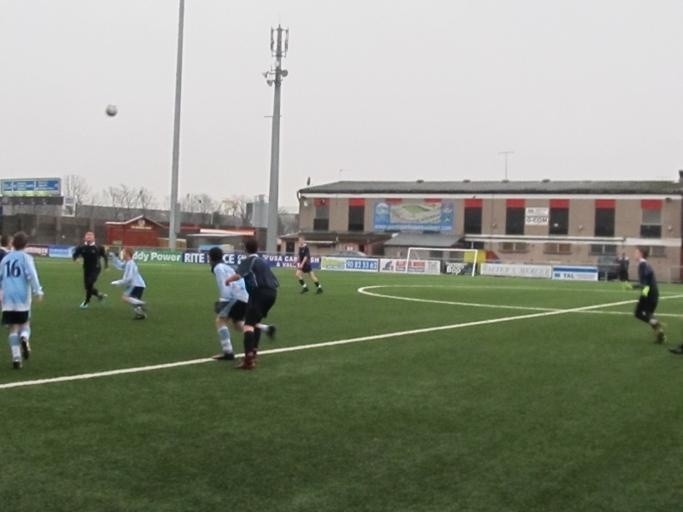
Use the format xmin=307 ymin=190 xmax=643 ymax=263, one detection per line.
xmin=72 ymin=231 xmax=106 ymax=309
xmin=667 ymin=343 xmax=682 ymax=356
xmin=615 ymin=252 xmax=629 ymax=282
xmin=619 ymin=244 xmax=670 ymax=344
xmin=294 ymin=235 xmax=323 ymax=294
xmin=0 ymin=232 xmax=43 ymax=369
xmin=224 ymin=240 xmax=278 ymax=369
xmin=107 ymin=247 xmax=147 ymax=321
xmin=208 ymin=247 xmax=275 ymax=359
xmin=0 ymin=235 xmax=13 ymax=261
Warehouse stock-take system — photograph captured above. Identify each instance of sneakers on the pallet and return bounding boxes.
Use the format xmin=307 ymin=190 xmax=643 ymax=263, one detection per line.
xmin=669 ymin=344 xmax=682 ymax=354
xmin=211 ymin=352 xmax=235 ymax=360
xmin=80 ymin=301 xmax=89 ymax=309
xmin=13 ymin=361 xmax=20 ymax=369
xmin=130 ymin=312 xmax=146 ymax=320
xmin=300 ymin=287 xmax=309 ymax=294
xmin=316 ymin=287 xmax=323 ymax=294
xmin=233 ymin=357 xmax=257 ymax=369
xmin=20 ymin=336 xmax=31 ymax=359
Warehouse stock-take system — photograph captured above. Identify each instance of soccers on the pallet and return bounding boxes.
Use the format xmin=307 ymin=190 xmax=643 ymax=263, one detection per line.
xmin=106 ymin=105 xmax=117 ymax=117
